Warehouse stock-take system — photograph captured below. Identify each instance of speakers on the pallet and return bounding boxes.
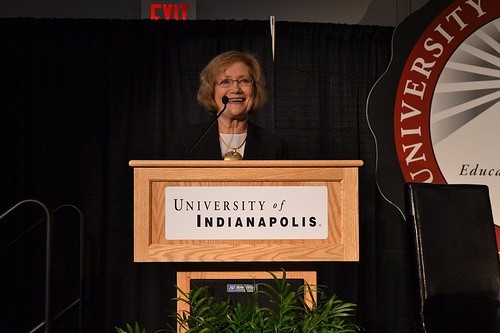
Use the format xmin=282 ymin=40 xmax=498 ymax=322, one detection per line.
xmin=404 ymin=181 xmax=500 ymax=333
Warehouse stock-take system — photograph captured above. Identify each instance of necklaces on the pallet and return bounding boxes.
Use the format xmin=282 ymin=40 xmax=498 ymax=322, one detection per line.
xmin=219 ymin=135 xmax=247 ymax=160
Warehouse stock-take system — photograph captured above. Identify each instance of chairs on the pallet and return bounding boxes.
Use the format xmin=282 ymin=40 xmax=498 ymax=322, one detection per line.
xmin=406 ymin=182 xmax=500 ymax=333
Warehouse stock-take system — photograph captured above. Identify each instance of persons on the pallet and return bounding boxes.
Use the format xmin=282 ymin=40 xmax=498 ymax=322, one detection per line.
xmin=170 ymin=50 xmax=291 ymax=159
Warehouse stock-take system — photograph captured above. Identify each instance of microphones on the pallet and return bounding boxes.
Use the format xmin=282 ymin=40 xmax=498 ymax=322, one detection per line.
xmin=184 ymin=95 xmax=229 ymax=160
